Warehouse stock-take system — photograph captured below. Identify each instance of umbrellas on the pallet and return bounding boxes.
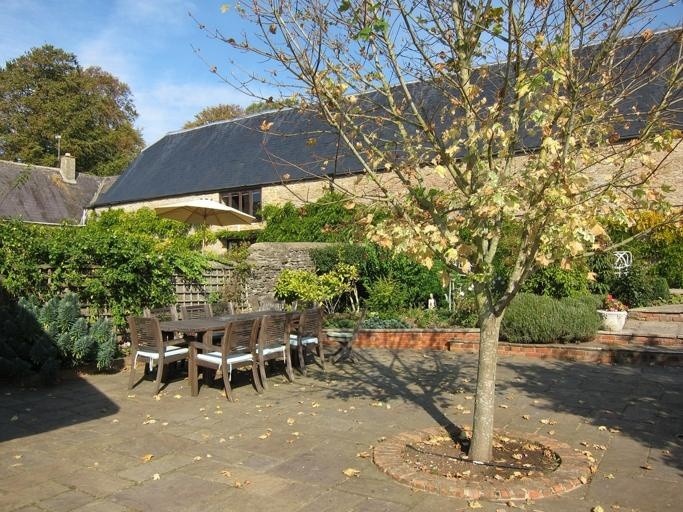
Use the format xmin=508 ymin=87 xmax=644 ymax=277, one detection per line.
xmin=154 ymin=199 xmax=256 ymax=255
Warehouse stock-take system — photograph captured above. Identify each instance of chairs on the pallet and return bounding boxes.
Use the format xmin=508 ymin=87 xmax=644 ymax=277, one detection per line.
xmin=128 ymin=301 xmax=325 ymax=404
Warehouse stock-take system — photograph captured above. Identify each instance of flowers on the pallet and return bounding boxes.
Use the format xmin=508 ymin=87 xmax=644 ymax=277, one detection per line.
xmin=605 ymin=295 xmax=630 ymax=312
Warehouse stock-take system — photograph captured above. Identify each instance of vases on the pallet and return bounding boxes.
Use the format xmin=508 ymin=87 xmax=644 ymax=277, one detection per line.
xmin=596 ymin=309 xmax=628 ymax=332
